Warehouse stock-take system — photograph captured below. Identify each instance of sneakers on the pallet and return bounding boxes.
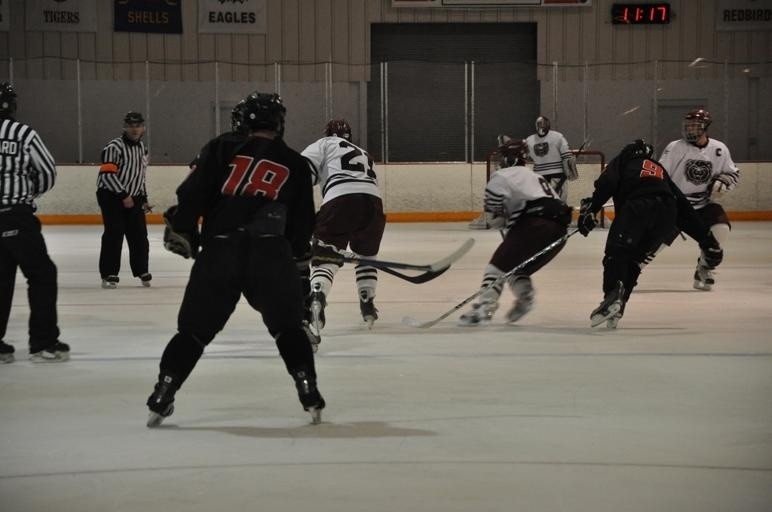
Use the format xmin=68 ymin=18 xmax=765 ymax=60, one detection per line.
xmin=102 ymin=274 xmax=119 ymax=283
xmin=590 ymin=292 xmax=618 ymax=320
xmin=606 ymin=303 xmax=625 ymax=330
xmin=0 ymin=341 xmax=16 ymax=353
xmin=147 ymin=383 xmax=175 ymax=417
xmin=29 ymin=340 xmax=69 ymax=354
xmin=506 ymin=292 xmax=536 ymax=324
xmin=358 ymin=289 xmax=378 ymax=321
xmin=140 ymin=273 xmax=152 ymax=281
xmin=291 ymin=368 xmax=325 ymax=411
xmin=460 ymin=298 xmax=499 ymax=321
xmin=693 ymin=270 xmax=714 ymax=285
xmin=309 ymin=291 xmax=327 ymax=329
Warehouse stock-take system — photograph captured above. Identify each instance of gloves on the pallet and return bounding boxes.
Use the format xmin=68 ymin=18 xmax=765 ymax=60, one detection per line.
xmin=706 ymin=178 xmax=727 ymax=204
xmin=576 ymin=197 xmax=599 ymax=236
xmin=162 ymin=206 xmax=198 ymax=260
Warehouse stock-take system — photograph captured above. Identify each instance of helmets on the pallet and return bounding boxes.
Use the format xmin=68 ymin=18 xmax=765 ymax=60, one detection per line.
xmin=683 ymin=110 xmax=711 ymax=142
xmin=324 ymin=119 xmax=352 ymax=143
xmin=496 ymin=139 xmax=530 ymax=168
xmin=0 ymin=81 xmax=16 ymax=98
xmin=623 ymin=139 xmax=654 ymax=158
xmin=123 ymin=111 xmax=145 ymax=124
xmin=231 ymin=90 xmax=287 ymax=137
xmin=535 ymin=117 xmax=550 ymax=137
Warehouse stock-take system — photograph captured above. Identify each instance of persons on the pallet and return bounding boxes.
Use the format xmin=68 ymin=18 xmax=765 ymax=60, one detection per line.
xmin=1 ymin=79 xmax=71 ymax=355
xmin=460 ymin=141 xmax=568 ymax=326
xmin=145 ymin=91 xmax=326 ymax=416
xmin=579 ymin=138 xmax=732 ymax=330
xmin=95 ymin=111 xmax=152 ymax=283
xmin=524 ymin=115 xmax=579 ymax=200
xmin=298 ymin=120 xmax=387 ymax=329
xmin=639 ymin=106 xmax=741 ymax=286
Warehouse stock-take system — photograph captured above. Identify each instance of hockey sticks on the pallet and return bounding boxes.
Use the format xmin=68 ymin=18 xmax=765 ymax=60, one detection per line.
xmin=402 ymin=229 xmax=580 ymax=329
xmin=338 ymin=249 xmax=451 ymax=284
xmin=311 ymin=238 xmax=475 ymax=271
